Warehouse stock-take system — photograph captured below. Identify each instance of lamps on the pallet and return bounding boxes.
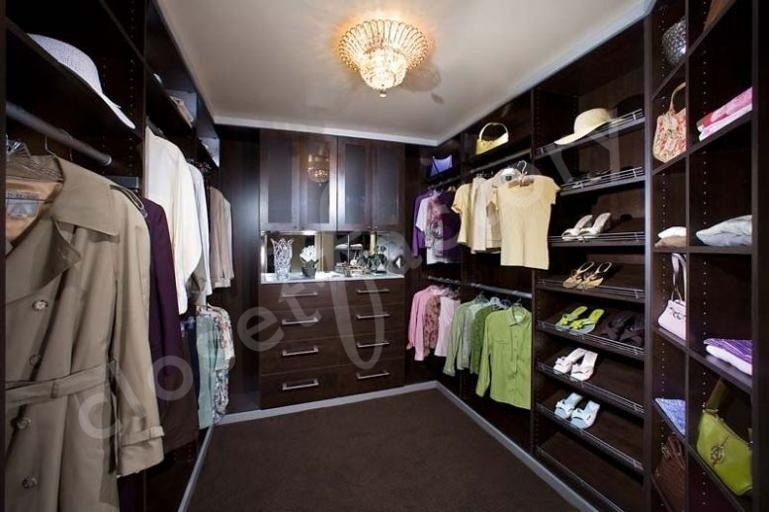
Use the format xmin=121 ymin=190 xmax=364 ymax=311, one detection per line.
xmin=338 ymin=15 xmax=428 ymax=101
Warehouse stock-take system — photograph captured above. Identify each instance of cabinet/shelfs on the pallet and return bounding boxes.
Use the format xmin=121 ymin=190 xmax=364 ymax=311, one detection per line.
xmin=0 ymin=1 xmax=223 ymax=512
xmin=254 ymin=129 xmax=434 ymax=411
xmin=427 ymin=1 xmax=766 ymax=512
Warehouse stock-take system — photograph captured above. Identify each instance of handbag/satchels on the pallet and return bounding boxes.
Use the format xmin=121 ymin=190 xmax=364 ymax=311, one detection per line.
xmin=695 ymin=403 xmax=753 ymax=497
xmin=430 ymin=154 xmax=453 ymax=177
xmin=653 ymin=434 xmax=687 ymax=512
xmin=651 ymin=81 xmax=687 ymax=164
xmin=475 ymin=122 xmax=509 ymax=155
xmin=657 ymin=252 xmax=687 ymax=341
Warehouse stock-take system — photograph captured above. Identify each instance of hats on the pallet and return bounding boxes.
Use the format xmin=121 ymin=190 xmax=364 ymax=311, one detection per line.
xmin=554 ymin=107 xmax=615 ymax=145
xmin=30 ymin=33 xmax=134 ymax=129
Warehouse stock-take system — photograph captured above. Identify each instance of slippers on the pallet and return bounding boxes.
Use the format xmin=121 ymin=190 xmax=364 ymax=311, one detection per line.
xmin=552 ymin=347 xmax=586 ymax=376
xmin=555 ymin=306 xmax=644 ymax=347
xmin=562 ymin=261 xmax=595 ymax=289
xmin=569 ymin=351 xmax=598 ymax=383
xmin=554 ymin=392 xmax=584 ymax=420
xmin=577 ymin=258 xmax=612 ymax=290
xmin=576 ymin=212 xmax=611 ymax=242
xmin=561 ymin=215 xmax=593 ymax=241
xmin=569 ymin=400 xmax=600 ymax=430
